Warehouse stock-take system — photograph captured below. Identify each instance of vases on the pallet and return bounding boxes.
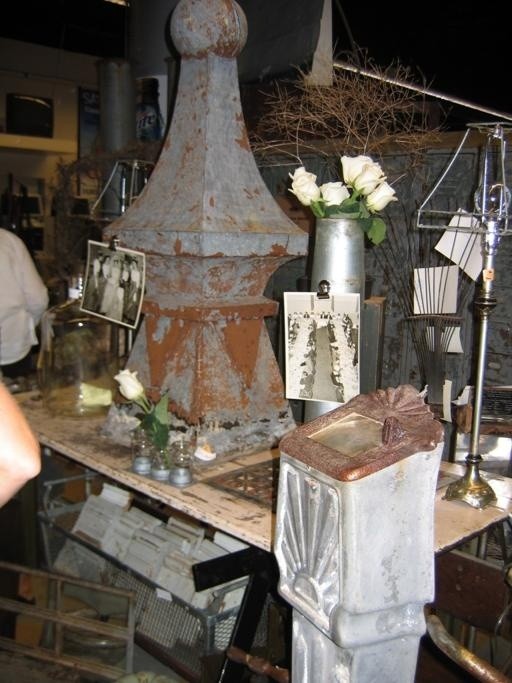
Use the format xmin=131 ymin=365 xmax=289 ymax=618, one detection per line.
xmin=150 ymin=443 xmax=173 ymax=480
xmin=304 ymin=218 xmax=365 ymax=422
xmin=170 ymin=441 xmax=192 ymax=488
xmin=130 ymin=431 xmax=154 ymax=475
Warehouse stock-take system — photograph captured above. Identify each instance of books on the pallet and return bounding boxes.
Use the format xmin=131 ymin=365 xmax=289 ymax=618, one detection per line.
xmin=50 ymin=481 xmax=248 ymax=651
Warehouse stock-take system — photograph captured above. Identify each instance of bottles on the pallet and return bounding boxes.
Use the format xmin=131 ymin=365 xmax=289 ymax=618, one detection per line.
xmin=131 ymin=429 xmax=153 ymax=476
xmin=170 ymin=435 xmax=195 ymax=487
xmin=38 ymin=274 xmax=119 ymax=418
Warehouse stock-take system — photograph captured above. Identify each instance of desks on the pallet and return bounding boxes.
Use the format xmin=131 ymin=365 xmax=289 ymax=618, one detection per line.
xmin=12 ymin=390 xmax=511 ymax=683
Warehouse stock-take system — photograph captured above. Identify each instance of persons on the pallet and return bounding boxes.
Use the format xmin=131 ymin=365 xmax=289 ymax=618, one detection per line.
xmin=0 ymin=226 xmax=47 ymax=379
xmin=82 ymin=252 xmax=141 ymax=321
xmin=288 ymin=311 xmax=359 ymax=402
xmin=0 ymin=380 xmax=42 ymax=510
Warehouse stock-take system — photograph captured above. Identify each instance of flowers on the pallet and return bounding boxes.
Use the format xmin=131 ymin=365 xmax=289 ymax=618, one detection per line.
xmin=288 ymin=155 xmax=399 ymax=243
xmin=114 ymin=370 xmax=172 ymax=467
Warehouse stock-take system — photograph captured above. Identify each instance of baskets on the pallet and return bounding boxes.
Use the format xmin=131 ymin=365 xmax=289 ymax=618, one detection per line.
xmin=39 ymin=501 xmax=272 ymax=681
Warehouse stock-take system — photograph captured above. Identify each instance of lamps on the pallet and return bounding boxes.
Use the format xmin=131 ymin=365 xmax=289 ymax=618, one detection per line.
xmin=90 ymin=159 xmax=155 ymax=223
xmin=417 ymin=122 xmax=512 ymax=510
